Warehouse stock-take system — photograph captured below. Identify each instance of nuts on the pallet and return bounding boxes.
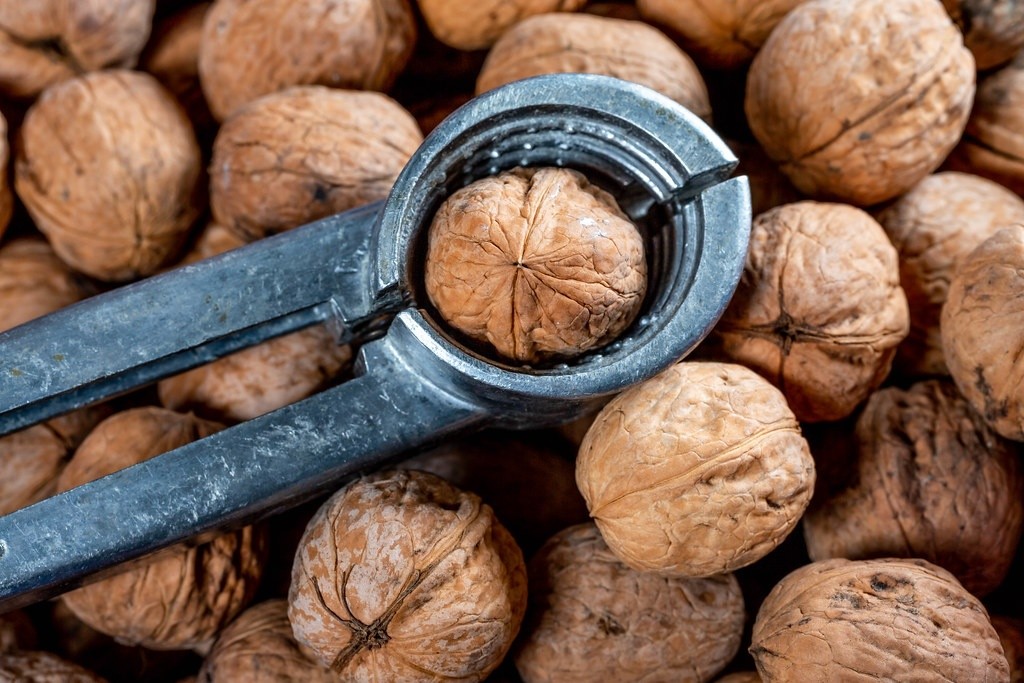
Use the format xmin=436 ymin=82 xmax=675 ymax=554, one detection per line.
xmin=1 ymin=0 xmax=1024 ymax=683
